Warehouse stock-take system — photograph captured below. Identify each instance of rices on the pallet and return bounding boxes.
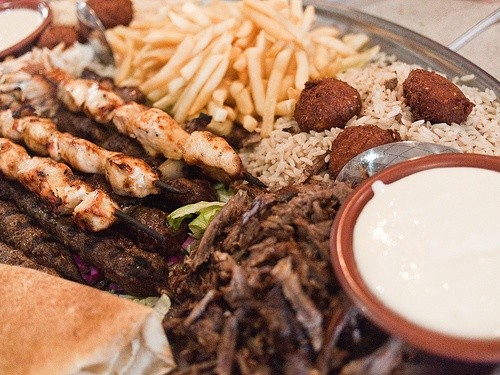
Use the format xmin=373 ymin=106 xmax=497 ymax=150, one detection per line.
xmin=238 ymin=59 xmax=500 ymax=186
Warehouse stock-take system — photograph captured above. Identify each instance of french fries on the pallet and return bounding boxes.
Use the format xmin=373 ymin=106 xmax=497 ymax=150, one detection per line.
xmin=105 ymin=0 xmax=381 ymax=139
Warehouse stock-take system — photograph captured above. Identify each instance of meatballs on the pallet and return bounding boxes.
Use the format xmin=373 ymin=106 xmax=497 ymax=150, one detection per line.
xmin=88 ymin=0 xmax=136 ymax=28
xmin=328 ymin=123 xmax=401 ymax=180
xmin=295 ymin=75 xmax=363 ymax=131
xmin=37 ymin=24 xmax=81 ymax=50
xmin=402 ymin=68 xmax=476 ymax=125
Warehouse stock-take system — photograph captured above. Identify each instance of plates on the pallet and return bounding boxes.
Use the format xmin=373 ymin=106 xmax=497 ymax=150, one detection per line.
xmin=329 ymin=149 xmax=500 ymax=368
xmin=0 ymin=0 xmax=50 ymax=62
